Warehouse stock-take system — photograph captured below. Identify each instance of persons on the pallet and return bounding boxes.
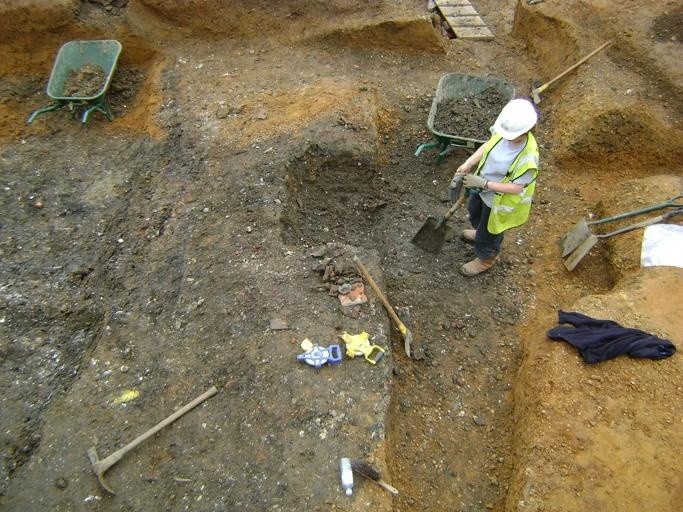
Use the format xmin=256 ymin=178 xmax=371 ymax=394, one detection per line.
xmin=446 ymin=97 xmax=541 ymax=280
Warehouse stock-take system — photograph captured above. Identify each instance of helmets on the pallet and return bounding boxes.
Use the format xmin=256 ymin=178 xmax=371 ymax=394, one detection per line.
xmin=493 ymin=98 xmax=538 ymax=141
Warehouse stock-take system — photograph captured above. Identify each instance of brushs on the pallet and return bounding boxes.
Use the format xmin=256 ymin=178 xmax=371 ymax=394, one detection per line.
xmin=350 ymin=459 xmax=399 ymax=495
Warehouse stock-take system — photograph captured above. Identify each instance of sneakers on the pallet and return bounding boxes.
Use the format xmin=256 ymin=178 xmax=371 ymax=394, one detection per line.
xmin=460 ymin=230 xmax=477 ymax=243
xmin=462 ymin=256 xmax=493 ymax=277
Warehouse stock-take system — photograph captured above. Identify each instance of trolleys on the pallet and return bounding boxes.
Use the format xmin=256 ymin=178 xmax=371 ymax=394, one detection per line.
xmin=413 ymin=72 xmax=517 ymax=161
xmin=26 ymin=38 xmax=122 ymax=125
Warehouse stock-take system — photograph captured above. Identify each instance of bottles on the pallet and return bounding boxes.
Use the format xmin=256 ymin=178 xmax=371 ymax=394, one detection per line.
xmin=341 ymin=458 xmax=353 ymax=496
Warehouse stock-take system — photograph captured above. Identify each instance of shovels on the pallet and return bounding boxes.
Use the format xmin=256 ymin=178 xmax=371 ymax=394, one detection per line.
xmin=557 ymin=195 xmax=683 ymax=259
xmin=410 ymin=186 xmax=482 ymax=258
xmin=561 ymin=206 xmax=683 ymax=272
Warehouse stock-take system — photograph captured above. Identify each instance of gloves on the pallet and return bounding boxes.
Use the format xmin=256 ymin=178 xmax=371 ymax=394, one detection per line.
xmin=463 ymin=173 xmax=488 ymax=189
xmin=448 ymin=172 xmax=465 ymax=204
